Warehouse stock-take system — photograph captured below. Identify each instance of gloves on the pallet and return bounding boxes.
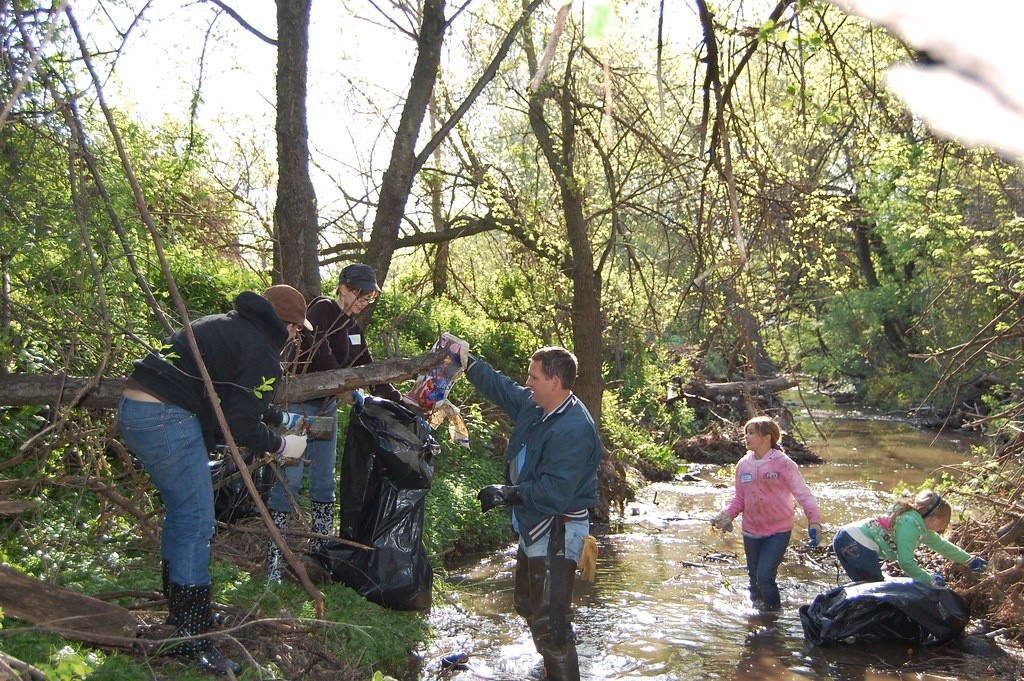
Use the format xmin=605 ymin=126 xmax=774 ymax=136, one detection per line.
xmin=461 ymin=353 xmax=478 ymax=370
xmin=714 ymin=509 xmax=732 ymax=529
xmin=477 ymin=484 xmax=523 ymax=514
xmin=965 ymin=554 xmax=988 ymax=574
xmin=283 ymin=411 xmax=312 ymax=433
xmin=336 ymin=389 xmax=358 ymax=405
xmin=808 ymin=522 xmax=822 ymax=547
xmin=276 ymin=434 xmax=308 ymax=459
xmin=931 ymin=573 xmax=946 ymax=587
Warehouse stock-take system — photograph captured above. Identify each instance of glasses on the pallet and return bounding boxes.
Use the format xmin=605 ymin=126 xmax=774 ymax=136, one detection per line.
xmin=346 ymin=285 xmax=375 ymax=304
xmin=287 ymin=322 xmax=303 ymax=331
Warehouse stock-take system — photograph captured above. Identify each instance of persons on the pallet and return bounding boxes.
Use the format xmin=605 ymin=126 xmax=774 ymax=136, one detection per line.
xmin=265 ymin=263 xmax=406 ymax=587
xmin=118 ymin=285 xmax=314 ymax=678
xmin=465 ymin=345 xmax=600 ymax=681
xmin=711 ymin=416 xmax=822 ymax=609
xmin=833 ymin=492 xmax=988 ymax=582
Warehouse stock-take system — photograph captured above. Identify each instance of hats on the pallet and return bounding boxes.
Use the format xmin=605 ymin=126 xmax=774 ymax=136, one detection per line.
xmin=339 ymin=264 xmax=383 ymax=293
xmin=264 ymin=284 xmax=313 ymax=332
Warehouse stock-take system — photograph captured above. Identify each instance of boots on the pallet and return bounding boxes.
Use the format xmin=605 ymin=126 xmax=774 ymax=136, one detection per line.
xmin=169 ymin=581 xmax=242 ymax=676
xmin=306 ymin=499 xmax=334 ymax=554
xmin=264 ymin=509 xmax=290 ymax=601
xmin=162 ymin=560 xmax=221 ymax=627
